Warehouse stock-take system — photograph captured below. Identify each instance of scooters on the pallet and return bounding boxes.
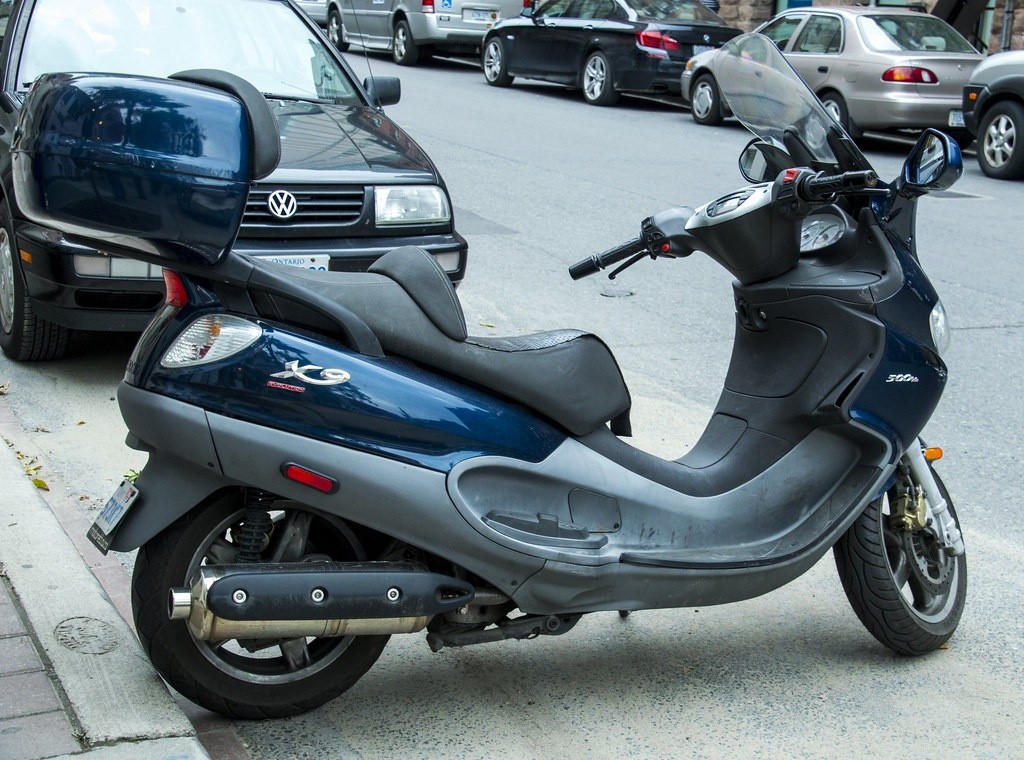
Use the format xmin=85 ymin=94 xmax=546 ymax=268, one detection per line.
xmin=87 ymin=24 xmax=970 ymax=724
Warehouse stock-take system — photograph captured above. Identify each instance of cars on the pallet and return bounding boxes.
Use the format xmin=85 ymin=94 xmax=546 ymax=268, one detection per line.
xmin=681 ymin=5 xmax=988 ymax=153
xmin=0 ymin=0 xmax=471 ymax=366
xmin=962 ymin=49 xmax=1024 ymax=182
xmin=292 ymin=0 xmax=545 ymax=67
xmin=483 ymin=0 xmax=751 ymax=109
